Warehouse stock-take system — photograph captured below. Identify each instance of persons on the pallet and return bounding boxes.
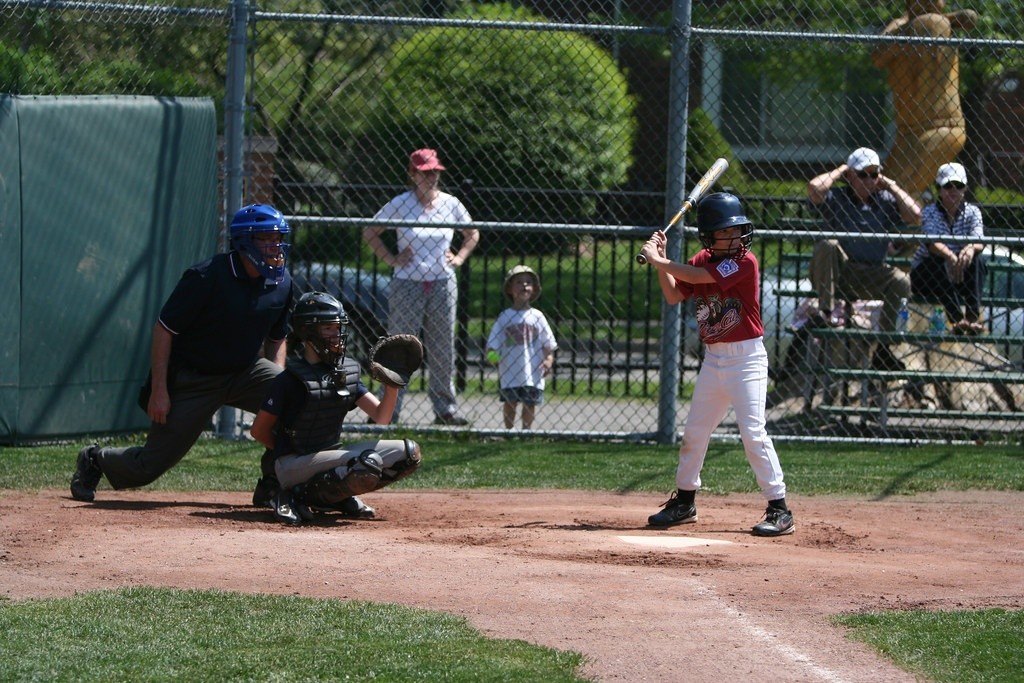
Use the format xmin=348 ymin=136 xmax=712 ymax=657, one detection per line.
xmin=250 ymin=291 xmax=422 ymax=522
xmin=909 ymin=161 xmax=987 ymax=336
xmin=360 ymin=148 xmax=479 ymax=425
xmin=485 ymin=266 xmax=558 ymax=430
xmin=640 ymin=193 xmax=796 ymax=536
xmin=809 ymin=147 xmax=922 ymax=371
xmin=875 ymin=0 xmax=977 ymax=274
xmin=69 ymin=198 xmax=292 ymax=507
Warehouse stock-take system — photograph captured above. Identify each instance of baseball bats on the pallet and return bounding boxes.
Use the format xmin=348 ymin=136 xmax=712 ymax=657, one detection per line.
xmin=637 ymin=157 xmax=729 ymax=265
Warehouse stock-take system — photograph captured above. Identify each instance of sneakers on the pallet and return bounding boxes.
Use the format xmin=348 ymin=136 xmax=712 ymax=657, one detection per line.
xmin=71 ymin=444 xmax=103 ymax=501
xmin=253 ymin=479 xmax=273 ymax=508
xmin=267 ymin=493 xmax=301 ymax=526
xmin=647 ymin=491 xmax=697 ymax=527
xmin=336 ymin=497 xmax=374 ymax=518
xmin=752 ymin=506 xmax=795 ymax=535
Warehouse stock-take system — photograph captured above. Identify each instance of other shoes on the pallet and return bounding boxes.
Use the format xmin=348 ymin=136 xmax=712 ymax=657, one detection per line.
xmin=818 ymin=313 xmax=839 ymax=327
xmin=954 ymin=319 xmax=983 ymax=334
xmin=434 ymin=411 xmax=468 ymax=425
xmin=872 ymin=350 xmax=904 ymax=371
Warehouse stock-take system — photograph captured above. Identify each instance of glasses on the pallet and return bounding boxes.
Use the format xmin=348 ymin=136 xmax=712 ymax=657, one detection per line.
xmin=943 ymin=182 xmax=965 ymax=189
xmin=853 ymin=169 xmax=879 ymax=179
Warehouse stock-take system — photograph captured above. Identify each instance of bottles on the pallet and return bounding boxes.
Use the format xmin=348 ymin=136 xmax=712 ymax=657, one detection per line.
xmin=929 ymin=307 xmax=944 ymax=335
xmin=895 ymin=297 xmax=909 ymax=332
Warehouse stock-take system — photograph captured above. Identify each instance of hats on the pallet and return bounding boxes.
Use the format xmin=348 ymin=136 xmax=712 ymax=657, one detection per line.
xmin=409 ymin=149 xmax=446 ymax=174
xmin=503 ymin=265 xmax=541 ymax=304
xmin=936 ymin=162 xmax=968 ymax=186
xmin=847 ymin=147 xmax=883 ymax=170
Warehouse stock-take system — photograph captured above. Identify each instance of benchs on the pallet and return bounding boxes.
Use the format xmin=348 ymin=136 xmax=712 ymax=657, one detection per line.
xmin=777 ymin=217 xmax=1024 ymax=436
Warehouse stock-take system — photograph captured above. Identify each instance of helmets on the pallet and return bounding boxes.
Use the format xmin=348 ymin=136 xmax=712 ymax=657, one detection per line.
xmin=230 ymin=203 xmax=289 ymax=282
xmin=291 ymin=291 xmax=348 ymax=368
xmin=696 ymin=192 xmax=753 ymax=258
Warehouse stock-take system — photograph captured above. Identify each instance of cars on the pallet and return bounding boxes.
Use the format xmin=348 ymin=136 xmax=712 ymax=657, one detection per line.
xmin=678 ymin=245 xmax=1023 ymax=386
xmin=285 ymin=260 xmax=394 ymax=374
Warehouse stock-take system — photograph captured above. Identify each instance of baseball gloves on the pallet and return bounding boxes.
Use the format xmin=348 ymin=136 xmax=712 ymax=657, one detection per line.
xmin=367 ymin=332 xmax=426 ymax=391
